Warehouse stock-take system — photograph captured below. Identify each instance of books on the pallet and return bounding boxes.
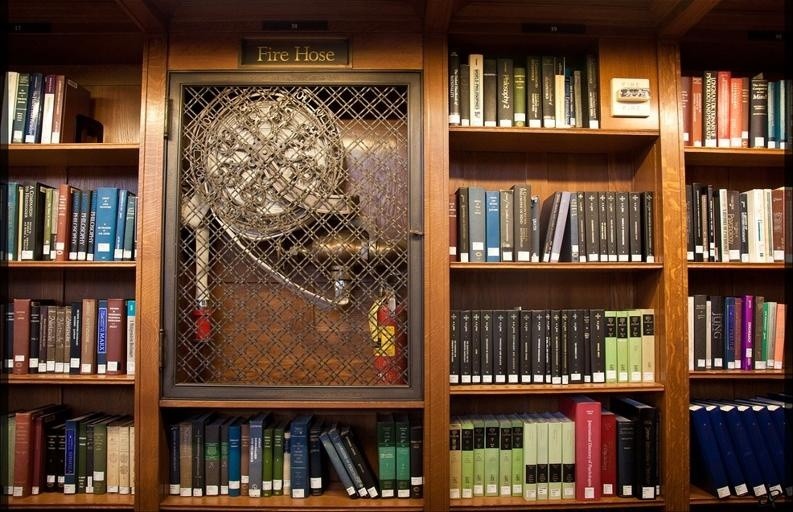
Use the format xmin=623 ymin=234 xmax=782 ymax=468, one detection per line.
xmin=689 ymin=295 xmax=792 ymax=373
xmin=166 ymin=410 xmax=422 ymax=499
xmin=1 ymin=71 xmax=97 ymax=144
xmin=448 ymin=44 xmax=599 ymax=128
xmin=687 ymin=179 xmax=792 ymax=267
xmin=448 ymin=308 xmax=656 ymax=384
xmin=690 ymin=396 xmax=792 ymax=501
xmin=1 ymin=298 xmax=135 ymax=376
xmin=683 ymin=70 xmax=791 ymax=155
xmin=449 ymin=184 xmax=655 ymax=263
xmin=1 ymin=181 xmax=137 ymax=263
xmin=1 ymin=403 xmax=139 ymax=498
xmin=447 ymin=396 xmax=664 ymax=502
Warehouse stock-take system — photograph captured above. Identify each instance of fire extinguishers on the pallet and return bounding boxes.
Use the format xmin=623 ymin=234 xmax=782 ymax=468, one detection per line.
xmin=369 ymin=275 xmax=407 ymax=386
